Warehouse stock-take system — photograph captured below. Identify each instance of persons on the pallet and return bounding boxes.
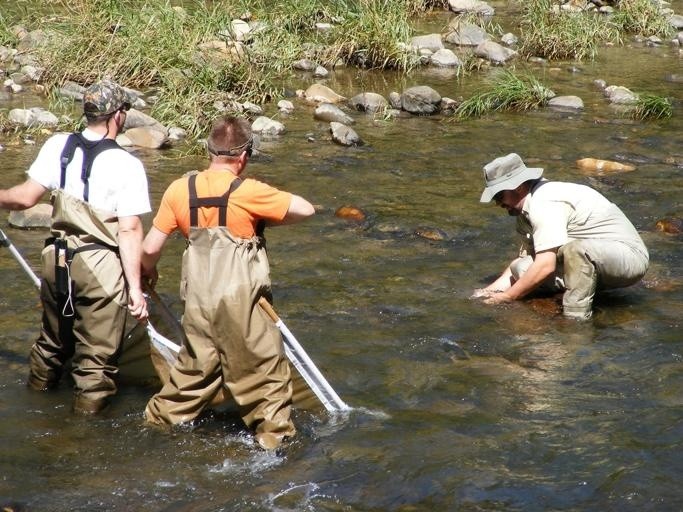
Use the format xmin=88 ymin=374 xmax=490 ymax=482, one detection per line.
xmin=465 ymin=149 xmax=649 ymax=325
xmin=1 ymin=80 xmax=153 ymax=418
xmin=136 ymin=113 xmax=316 ymax=457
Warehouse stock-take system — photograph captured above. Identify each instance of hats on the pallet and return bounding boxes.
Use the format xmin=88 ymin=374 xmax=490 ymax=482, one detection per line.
xmin=85 ymin=81 xmax=131 ymax=116
xmin=477 ymin=152 xmax=545 ymax=204
